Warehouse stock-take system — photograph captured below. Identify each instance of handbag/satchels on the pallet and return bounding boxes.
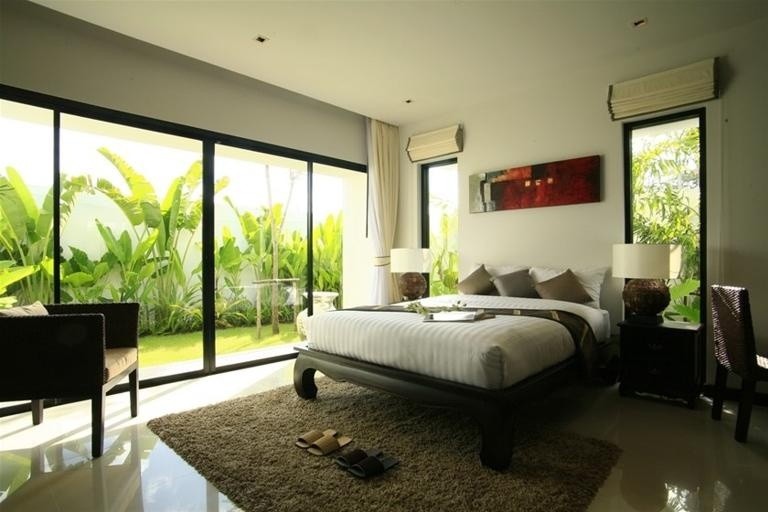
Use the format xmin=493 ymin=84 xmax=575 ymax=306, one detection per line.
xmin=423 ymin=312 xmax=495 ymax=322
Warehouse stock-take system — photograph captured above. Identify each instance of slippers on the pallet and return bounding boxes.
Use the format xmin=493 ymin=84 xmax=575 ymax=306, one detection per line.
xmin=335 ymin=447 xmax=382 ymax=468
xmin=295 ymin=429 xmax=337 ymax=449
xmin=307 ymin=434 xmax=353 ymax=456
xmin=348 ymin=456 xmax=399 ymax=479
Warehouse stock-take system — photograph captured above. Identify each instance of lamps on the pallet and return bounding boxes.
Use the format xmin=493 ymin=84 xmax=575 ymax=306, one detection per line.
xmin=389 ymin=248 xmax=434 ymax=301
xmin=611 ymin=243 xmax=680 ymax=325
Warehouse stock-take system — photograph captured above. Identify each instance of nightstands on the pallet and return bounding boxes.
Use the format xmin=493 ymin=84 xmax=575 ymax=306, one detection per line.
xmin=615 ymin=321 xmax=706 ymax=409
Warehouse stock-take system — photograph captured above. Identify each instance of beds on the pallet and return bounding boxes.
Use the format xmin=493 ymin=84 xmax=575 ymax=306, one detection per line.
xmin=294 ymin=265 xmax=623 ymax=473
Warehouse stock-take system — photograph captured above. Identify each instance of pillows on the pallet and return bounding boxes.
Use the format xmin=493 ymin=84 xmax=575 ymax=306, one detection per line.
xmin=457 ymin=264 xmax=495 ymax=295
xmin=531 ymin=267 xmax=608 ymax=308
xmin=0 ymin=300 xmax=49 ymax=316
xmin=530 ymin=268 xmax=593 ymax=304
xmin=489 ymin=269 xmax=538 ymax=298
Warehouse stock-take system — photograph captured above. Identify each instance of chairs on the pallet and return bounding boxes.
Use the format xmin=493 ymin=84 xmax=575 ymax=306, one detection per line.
xmin=0 ymin=421 xmax=142 ymax=512
xmin=0 ymin=302 xmax=139 ymax=458
xmin=711 ymin=285 xmax=767 ymax=443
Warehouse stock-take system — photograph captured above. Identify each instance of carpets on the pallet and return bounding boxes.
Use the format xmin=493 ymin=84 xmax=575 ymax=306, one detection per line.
xmin=147 ymin=371 xmax=623 ymax=510
